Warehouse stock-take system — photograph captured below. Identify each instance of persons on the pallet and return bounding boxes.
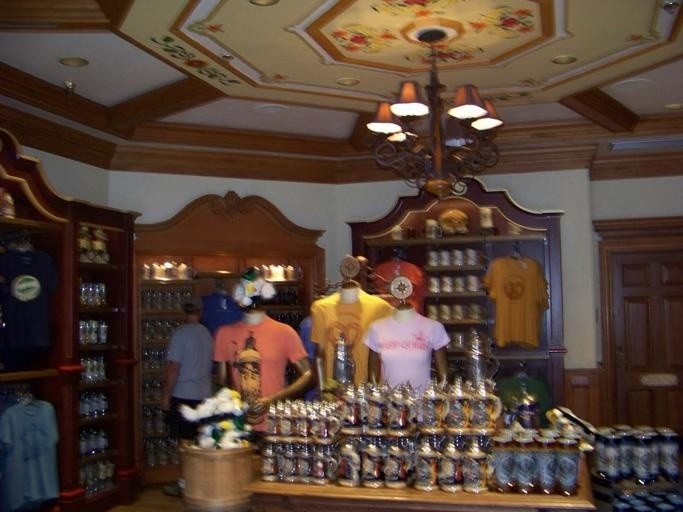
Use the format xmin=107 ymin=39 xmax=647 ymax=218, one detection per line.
xmin=357 ymin=305 xmax=450 ymax=391
xmin=310 ymin=281 xmax=393 ymax=401
xmin=156 ymin=303 xmax=213 ymax=495
xmin=211 ymin=308 xmax=313 ymax=442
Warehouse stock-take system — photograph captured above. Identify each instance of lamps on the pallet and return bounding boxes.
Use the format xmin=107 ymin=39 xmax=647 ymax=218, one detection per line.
xmin=365 ymin=44 xmax=505 ymax=197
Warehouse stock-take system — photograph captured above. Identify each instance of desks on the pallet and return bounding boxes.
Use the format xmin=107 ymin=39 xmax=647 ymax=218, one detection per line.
xmin=244 ymin=451 xmax=597 ymax=512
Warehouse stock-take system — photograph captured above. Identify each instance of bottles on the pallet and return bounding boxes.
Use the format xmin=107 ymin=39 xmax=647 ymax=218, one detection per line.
xmin=79 ymin=430 xmax=110 ymax=456
xmin=80 ymin=392 xmax=109 ymax=417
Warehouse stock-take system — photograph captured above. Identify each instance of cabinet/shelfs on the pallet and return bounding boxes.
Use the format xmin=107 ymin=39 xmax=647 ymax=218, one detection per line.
xmin=344 ymin=175 xmax=568 ymax=412
xmin=0 ymin=126 xmax=143 ymax=511
xmin=136 ymin=189 xmax=329 ymax=489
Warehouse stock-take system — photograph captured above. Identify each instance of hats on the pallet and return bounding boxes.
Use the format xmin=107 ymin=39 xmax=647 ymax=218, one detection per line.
xmin=183 ymin=295 xmax=203 ymax=311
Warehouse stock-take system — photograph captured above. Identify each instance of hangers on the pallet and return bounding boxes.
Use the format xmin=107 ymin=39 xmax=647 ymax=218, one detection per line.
xmin=391 ymin=247 xmax=402 ymax=262
xmin=517 ymin=362 xmax=529 ymax=380
xmin=511 ymin=239 xmax=522 ymax=260
xmin=0 ymin=382 xmax=35 ymax=407
xmin=0 ymin=228 xmax=36 ymax=252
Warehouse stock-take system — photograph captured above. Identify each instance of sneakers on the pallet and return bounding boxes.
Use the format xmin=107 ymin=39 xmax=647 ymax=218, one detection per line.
xmin=163 ymin=485 xmax=182 ymax=497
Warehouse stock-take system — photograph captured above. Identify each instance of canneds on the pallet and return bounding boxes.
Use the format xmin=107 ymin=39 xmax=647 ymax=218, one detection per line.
xmin=610 ymin=489 xmax=682 ymax=508
xmin=493 ymin=427 xmax=584 ymax=495
xmin=593 ymin=423 xmax=680 ymax=485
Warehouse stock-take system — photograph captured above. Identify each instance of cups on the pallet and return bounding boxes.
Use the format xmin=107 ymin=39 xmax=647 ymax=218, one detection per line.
xmin=425 ymin=219 xmax=442 ymax=239
